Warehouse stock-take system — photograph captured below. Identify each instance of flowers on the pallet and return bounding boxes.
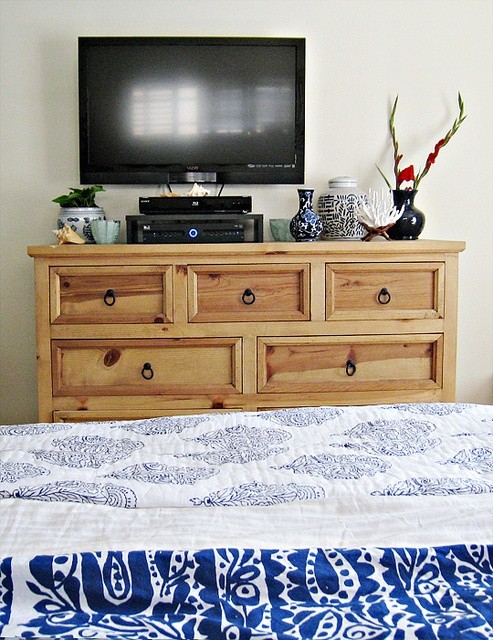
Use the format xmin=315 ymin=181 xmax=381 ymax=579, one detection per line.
xmin=375 ymin=90 xmax=467 ymax=192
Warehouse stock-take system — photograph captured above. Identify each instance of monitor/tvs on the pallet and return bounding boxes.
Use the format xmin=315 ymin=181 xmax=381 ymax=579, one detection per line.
xmin=78 ymin=36 xmax=306 ymax=185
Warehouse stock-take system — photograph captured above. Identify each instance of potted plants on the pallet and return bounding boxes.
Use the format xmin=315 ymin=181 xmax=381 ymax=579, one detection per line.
xmin=52 ymin=185 xmax=105 ymax=243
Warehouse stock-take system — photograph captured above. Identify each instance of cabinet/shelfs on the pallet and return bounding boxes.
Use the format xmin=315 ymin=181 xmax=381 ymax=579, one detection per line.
xmin=28 ymin=242 xmax=465 ymax=424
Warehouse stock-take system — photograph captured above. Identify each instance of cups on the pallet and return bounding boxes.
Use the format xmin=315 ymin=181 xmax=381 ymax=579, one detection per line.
xmin=90 ymin=220 xmax=121 ymax=246
xmin=269 ymin=218 xmax=297 ymax=242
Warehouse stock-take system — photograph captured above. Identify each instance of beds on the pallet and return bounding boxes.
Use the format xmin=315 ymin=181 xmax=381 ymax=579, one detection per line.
xmin=0 ymin=403 xmax=493 ymax=640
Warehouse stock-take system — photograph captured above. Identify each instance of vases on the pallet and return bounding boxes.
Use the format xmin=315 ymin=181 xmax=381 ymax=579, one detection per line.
xmin=386 ymin=190 xmax=425 ymax=240
xmin=289 ymin=189 xmax=323 ymax=242
